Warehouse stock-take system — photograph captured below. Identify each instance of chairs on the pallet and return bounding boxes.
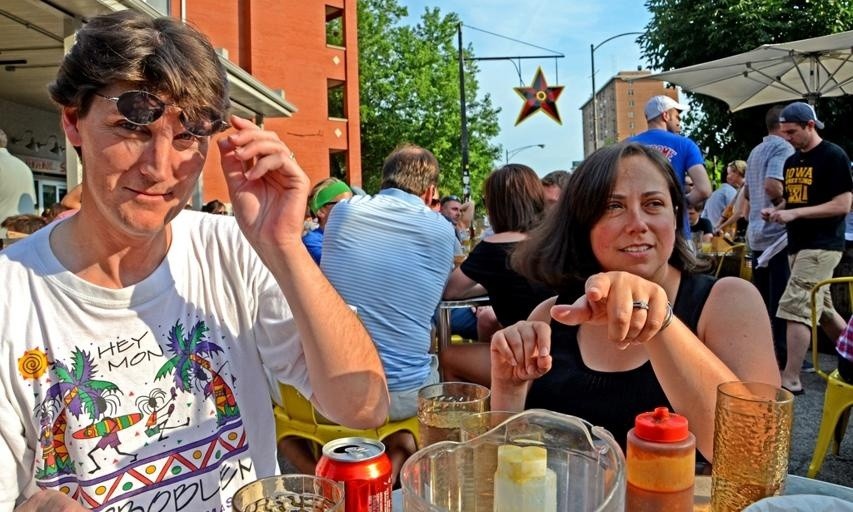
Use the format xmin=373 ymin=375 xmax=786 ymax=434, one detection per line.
xmin=274 ymin=303 xmax=422 ymax=459
xmin=808 ymin=278 xmax=852 ymax=480
xmin=713 ymin=242 xmax=747 ymax=277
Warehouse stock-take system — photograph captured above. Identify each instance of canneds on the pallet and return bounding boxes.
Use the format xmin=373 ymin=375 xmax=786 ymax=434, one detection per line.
xmin=314 ymin=436 xmax=393 ymax=512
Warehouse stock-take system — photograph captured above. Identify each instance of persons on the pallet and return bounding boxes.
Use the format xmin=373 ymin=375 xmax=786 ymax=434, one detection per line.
xmin=2 ymin=182 xmax=86 ymax=249
xmin=758 ymin=101 xmax=853 ymax=395
xmin=836 ymin=314 xmax=853 ymax=389
xmin=622 ymin=93 xmax=713 ymax=261
xmin=202 ymin=139 xmax=571 ymax=421
xmin=1 ymin=9 xmax=393 ymax=512
xmin=0 ymin=127 xmax=38 ymax=239
xmin=688 ymin=160 xmax=747 ymax=243
xmin=745 ymin=103 xmax=816 ymax=375
xmin=485 ymin=141 xmax=784 ymax=474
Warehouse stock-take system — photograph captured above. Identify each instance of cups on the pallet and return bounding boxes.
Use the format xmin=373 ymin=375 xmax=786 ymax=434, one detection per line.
xmin=227 ymin=474 xmax=343 ymax=512
xmin=691 ymin=229 xmax=702 ymax=255
xmin=460 ymin=409 xmax=545 ymax=512
xmin=416 ymin=381 xmax=492 ymax=448
xmin=708 ymin=383 xmax=794 ymax=511
xmin=458 ymin=216 xmax=484 ymax=253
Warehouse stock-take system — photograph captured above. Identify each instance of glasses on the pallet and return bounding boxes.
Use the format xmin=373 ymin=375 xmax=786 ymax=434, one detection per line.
xmin=93 ymin=90 xmax=232 ymax=137
xmin=448 ymin=196 xmax=461 ymax=201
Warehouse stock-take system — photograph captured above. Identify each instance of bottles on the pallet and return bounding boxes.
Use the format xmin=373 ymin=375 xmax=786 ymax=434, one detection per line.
xmin=625 ymin=402 xmax=695 ymax=512
xmin=494 ymin=446 xmax=558 ymax=512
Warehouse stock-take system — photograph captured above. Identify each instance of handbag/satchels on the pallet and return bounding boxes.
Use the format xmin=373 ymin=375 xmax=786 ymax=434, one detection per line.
xmin=717 ymin=203 xmax=738 ymax=234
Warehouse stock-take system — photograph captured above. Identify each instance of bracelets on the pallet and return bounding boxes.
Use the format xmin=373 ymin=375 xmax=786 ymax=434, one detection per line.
xmin=659 ymin=301 xmax=674 ymax=332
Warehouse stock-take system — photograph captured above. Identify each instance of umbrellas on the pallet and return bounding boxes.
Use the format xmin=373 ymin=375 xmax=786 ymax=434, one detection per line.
xmin=622 ymin=26 xmax=853 ymax=122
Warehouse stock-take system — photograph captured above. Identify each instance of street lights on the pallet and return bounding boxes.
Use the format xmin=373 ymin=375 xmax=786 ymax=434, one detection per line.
xmin=504 ymin=144 xmax=546 ymax=164
xmin=589 ymin=30 xmax=661 ymax=151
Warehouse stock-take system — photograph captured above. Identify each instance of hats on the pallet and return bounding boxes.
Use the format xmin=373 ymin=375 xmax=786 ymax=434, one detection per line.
xmin=644 ymin=96 xmax=690 ymax=120
xmin=779 ymin=102 xmax=824 ymax=130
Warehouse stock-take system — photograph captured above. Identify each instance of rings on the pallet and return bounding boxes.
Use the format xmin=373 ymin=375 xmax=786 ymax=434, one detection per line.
xmin=633 ymin=298 xmax=650 ymax=310
xmin=289 ymin=151 xmax=295 ymax=161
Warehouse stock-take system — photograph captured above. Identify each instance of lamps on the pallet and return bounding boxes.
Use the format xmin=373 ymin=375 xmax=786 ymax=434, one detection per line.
xmin=36 ymin=135 xmax=61 ymax=156
xmin=8 ymin=129 xmax=38 ymax=154
